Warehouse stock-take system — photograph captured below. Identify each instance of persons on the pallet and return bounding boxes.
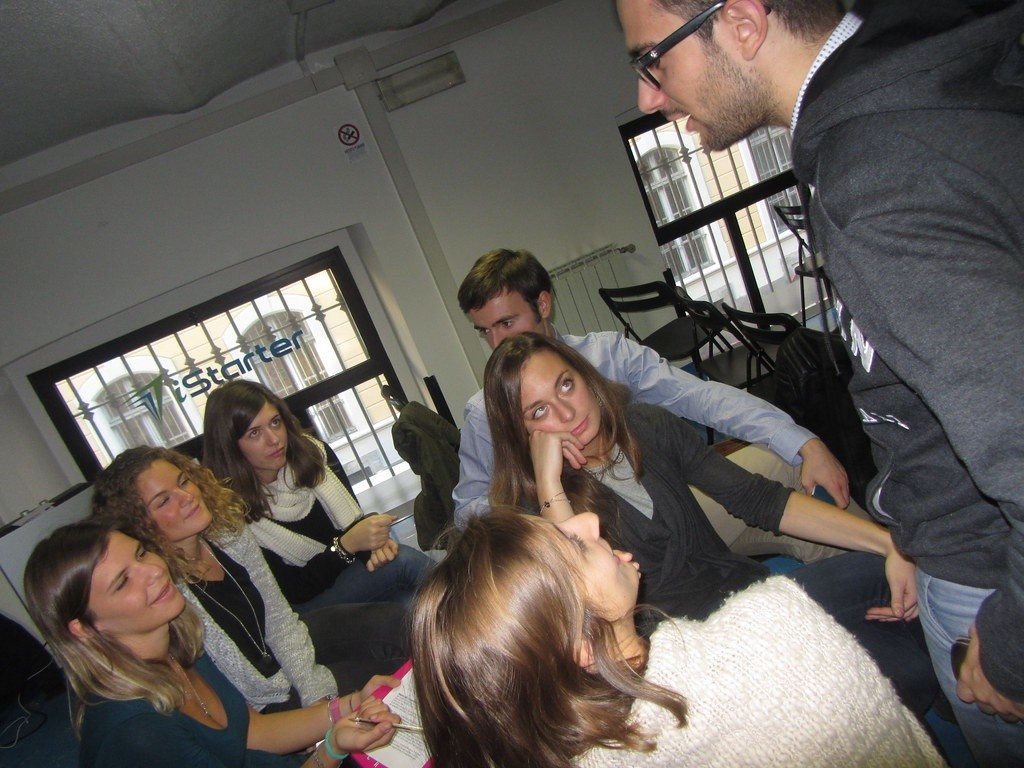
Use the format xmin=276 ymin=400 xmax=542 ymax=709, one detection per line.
xmin=405 ymin=502 xmax=948 ymax=768
xmin=22 ymin=519 xmax=403 ymax=768
xmin=483 ymin=329 xmax=960 ymax=725
xmin=89 ymin=445 xmax=410 ymax=755
xmin=610 ymin=1 xmax=1024 ymax=768
xmin=201 ymin=380 xmax=439 ymax=614
xmin=450 ymin=248 xmax=884 ymax=565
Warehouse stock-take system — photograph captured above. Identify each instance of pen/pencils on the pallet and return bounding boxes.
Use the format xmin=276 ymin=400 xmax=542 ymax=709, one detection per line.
xmin=347 ymin=718 xmax=425 ymax=732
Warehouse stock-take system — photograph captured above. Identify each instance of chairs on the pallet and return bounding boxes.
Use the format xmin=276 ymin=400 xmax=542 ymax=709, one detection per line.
xmin=671 ymin=286 xmax=781 ymax=389
xmin=599 ymin=281 xmax=732 ymax=379
xmin=722 ymin=303 xmax=801 ymax=369
xmin=775 ymin=206 xmax=840 ymax=330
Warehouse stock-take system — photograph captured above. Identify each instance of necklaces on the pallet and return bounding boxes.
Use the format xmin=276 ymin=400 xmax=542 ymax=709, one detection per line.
xmin=163 ymin=654 xmax=209 ymax=717
xmin=185 ymin=538 xmax=272 ymax=664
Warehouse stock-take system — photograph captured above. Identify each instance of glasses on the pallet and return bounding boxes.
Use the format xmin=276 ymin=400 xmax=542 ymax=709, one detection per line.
xmin=629 ymin=0 xmax=772 ymax=92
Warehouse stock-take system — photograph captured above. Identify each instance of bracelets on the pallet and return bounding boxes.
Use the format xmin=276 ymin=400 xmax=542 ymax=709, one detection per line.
xmin=330 ymin=536 xmax=355 ymax=565
xmin=322 ymin=694 xmax=338 ymax=700
xmin=328 ymin=697 xmax=342 ymax=726
xmin=349 ymin=691 xmax=355 ymax=713
xmin=539 ymin=491 xmax=565 ymax=516
xmin=325 ymin=727 xmax=350 ymax=760
